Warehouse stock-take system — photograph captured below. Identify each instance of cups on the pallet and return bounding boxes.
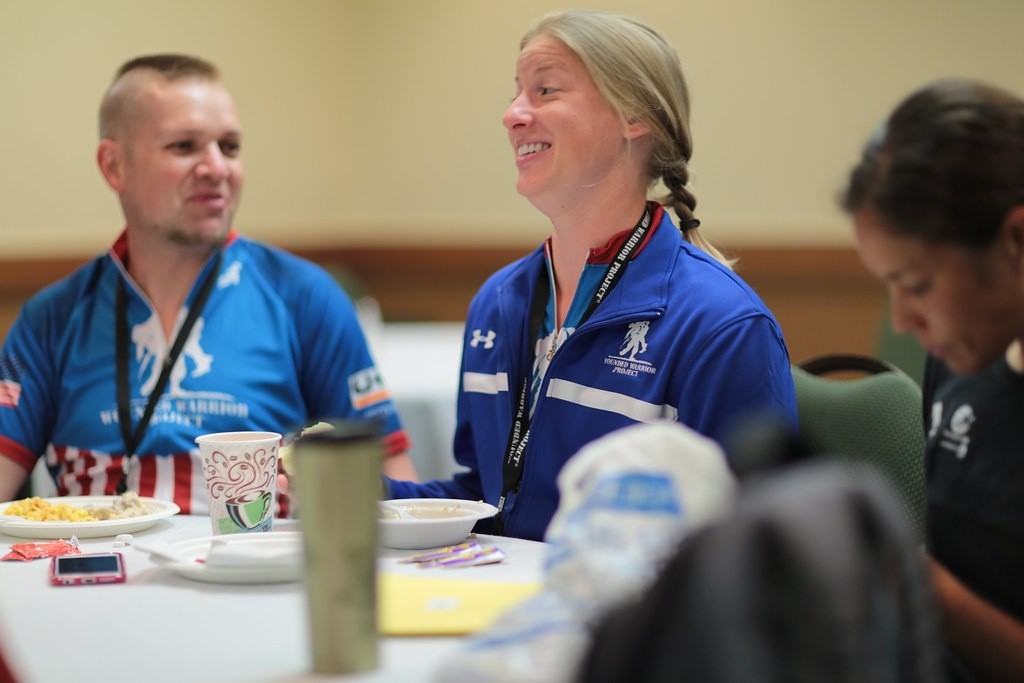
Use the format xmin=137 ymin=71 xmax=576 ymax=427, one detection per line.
xmin=195 ymin=430 xmax=282 ymax=536
xmin=289 ymin=419 xmax=387 ymax=676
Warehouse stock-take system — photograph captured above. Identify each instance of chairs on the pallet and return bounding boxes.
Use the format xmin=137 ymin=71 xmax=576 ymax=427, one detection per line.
xmin=789 ymin=351 xmax=925 ymax=554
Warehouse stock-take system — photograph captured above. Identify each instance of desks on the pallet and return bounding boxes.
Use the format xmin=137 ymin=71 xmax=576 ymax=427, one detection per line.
xmin=0 ymin=505 xmax=552 ymax=683
xmin=368 ymin=323 xmax=470 ymax=482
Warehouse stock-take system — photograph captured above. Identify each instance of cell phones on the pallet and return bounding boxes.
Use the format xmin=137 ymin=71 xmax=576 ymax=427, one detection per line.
xmin=49 ymin=552 xmax=126 ymax=585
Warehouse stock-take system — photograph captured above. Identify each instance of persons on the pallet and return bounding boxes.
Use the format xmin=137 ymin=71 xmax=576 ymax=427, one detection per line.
xmin=0 ymin=54 xmax=419 ymax=515
xmin=836 ymin=79 xmax=1024 ymax=683
xmin=379 ymin=12 xmax=800 ymax=544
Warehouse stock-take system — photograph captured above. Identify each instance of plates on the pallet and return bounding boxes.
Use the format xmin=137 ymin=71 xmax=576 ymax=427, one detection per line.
xmin=0 ymin=495 xmax=181 ymax=539
xmin=376 ymin=497 xmax=500 ymax=550
xmin=158 ymin=531 xmax=305 ymax=584
